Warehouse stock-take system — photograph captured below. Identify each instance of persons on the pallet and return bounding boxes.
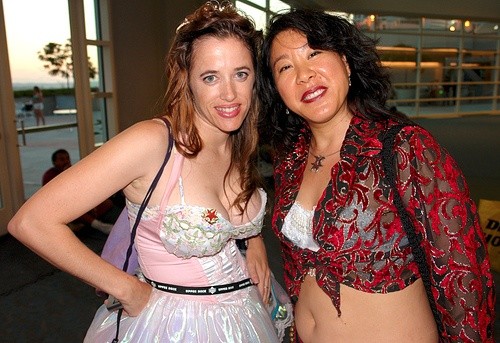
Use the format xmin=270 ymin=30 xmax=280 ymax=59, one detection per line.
xmin=32 ymin=86 xmax=46 ymax=125
xmin=6 ymin=0 xmax=280 ymax=343
xmin=40 ymin=149 xmax=114 ymax=233
xmin=243 ymin=9 xmax=500 ymax=343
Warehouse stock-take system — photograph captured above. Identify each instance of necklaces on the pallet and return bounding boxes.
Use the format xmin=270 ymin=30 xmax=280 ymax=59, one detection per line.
xmin=309 ymin=151 xmax=341 ymax=172
